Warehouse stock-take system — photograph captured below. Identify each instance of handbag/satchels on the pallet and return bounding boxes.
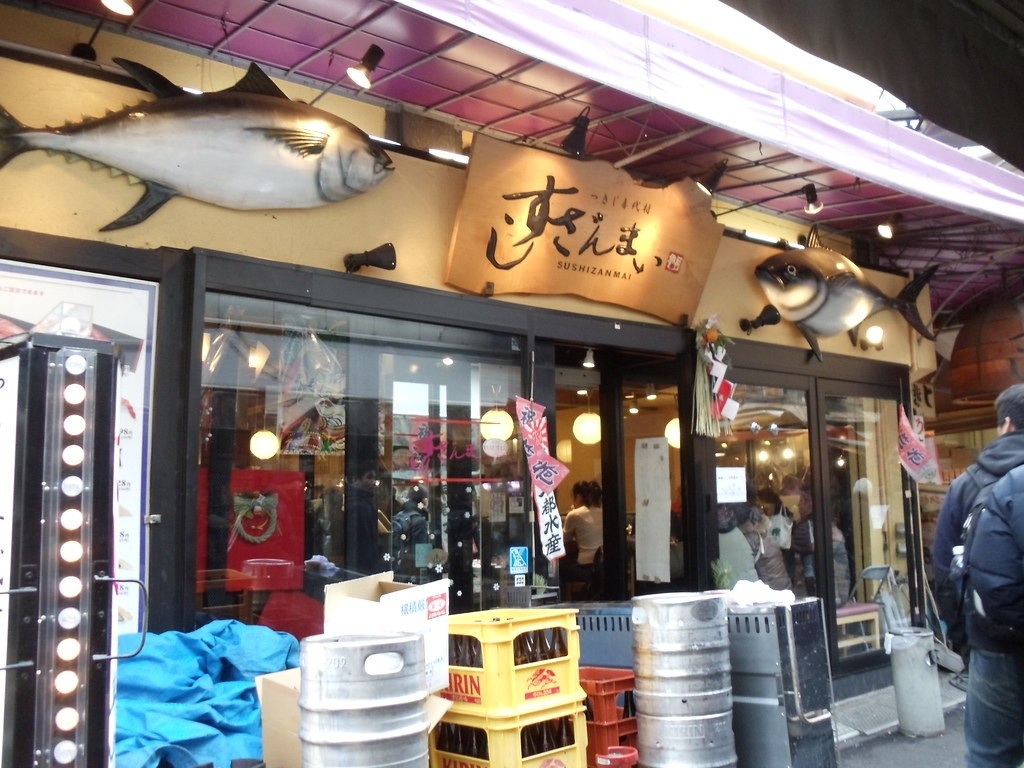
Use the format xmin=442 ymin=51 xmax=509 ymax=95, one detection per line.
xmin=770 ymin=502 xmax=793 ymax=550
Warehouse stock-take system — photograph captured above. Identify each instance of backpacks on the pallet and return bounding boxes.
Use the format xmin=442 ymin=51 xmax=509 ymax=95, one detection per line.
xmin=392 ymin=510 xmax=422 ymax=569
xmin=949 ymin=463 xmax=1024 ymax=660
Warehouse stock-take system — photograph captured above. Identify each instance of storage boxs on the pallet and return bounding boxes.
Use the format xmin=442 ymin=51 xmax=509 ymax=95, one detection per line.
xmin=256 ymin=571 xmax=639 ymax=768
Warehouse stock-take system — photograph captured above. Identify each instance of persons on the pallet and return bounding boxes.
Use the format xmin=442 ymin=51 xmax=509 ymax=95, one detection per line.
xmin=309 ymin=461 xmax=432 ymax=582
xmin=558 ymin=481 xmax=603 ymax=583
xmin=932 ymin=382 xmax=1024 ymax=768
xmin=716 ymin=474 xmax=851 ymax=608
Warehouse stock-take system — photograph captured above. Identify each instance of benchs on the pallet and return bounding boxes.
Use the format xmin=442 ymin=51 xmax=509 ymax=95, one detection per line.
xmin=836 ymin=602 xmax=882 ymax=651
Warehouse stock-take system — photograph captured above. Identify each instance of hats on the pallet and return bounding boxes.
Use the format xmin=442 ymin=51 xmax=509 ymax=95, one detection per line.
xmin=408 ymin=485 xmax=428 ymax=503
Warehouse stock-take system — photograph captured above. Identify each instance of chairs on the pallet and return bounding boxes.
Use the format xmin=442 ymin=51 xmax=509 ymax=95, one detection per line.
xmin=838 ymin=564 xmax=891 ymax=651
xmin=560 ymin=538 xmax=635 ymax=602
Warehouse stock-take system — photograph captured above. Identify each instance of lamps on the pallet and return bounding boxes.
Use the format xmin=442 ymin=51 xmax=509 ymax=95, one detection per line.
xmin=249 ymin=413 xmax=280 ymax=460
xmin=739 ymin=304 xmax=781 ymax=336
xmin=347 ymin=44 xmax=385 ymax=90
xmin=557 ymin=438 xmax=573 ymax=463
xmin=343 ymin=242 xmax=396 ymax=275
xmin=878 ymin=212 xmax=903 ymax=239
xmin=570 ymin=387 xmax=603 ymax=445
xmin=665 ymin=394 xmax=685 ymax=450
xmin=582 ymin=346 xmax=597 ymax=368
xmin=696 ymin=158 xmax=728 ymax=197
xmin=101 ymin=0 xmax=137 ymax=16
xmin=859 ymin=325 xmax=885 ymax=352
xmin=479 ymin=385 xmax=515 ymax=441
xmin=803 ymin=183 xmax=824 ymax=215
xmin=560 ymin=106 xmax=591 ymax=157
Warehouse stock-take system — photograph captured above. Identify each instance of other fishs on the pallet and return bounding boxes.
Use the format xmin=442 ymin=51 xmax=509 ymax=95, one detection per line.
xmin=1 ymin=57 xmax=396 ymax=235
xmin=754 ymin=223 xmax=940 ymax=363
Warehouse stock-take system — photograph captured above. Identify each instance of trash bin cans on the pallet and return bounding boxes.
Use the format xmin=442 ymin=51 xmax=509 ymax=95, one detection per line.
xmin=885 ymin=625 xmax=947 ymax=743
